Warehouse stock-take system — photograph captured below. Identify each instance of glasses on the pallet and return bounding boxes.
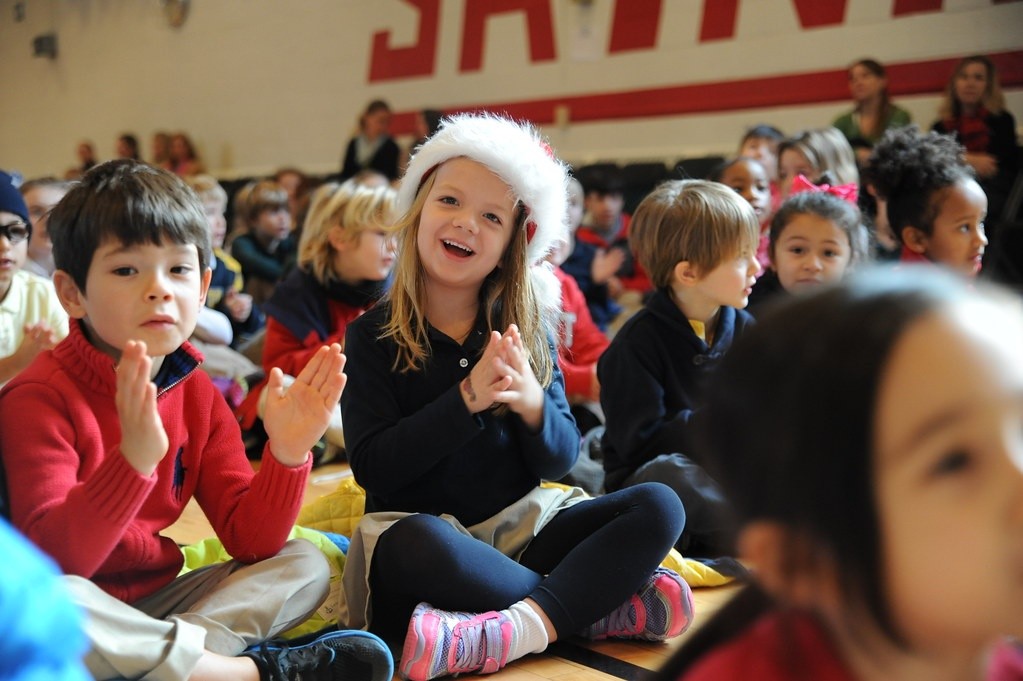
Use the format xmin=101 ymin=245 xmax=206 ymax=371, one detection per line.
xmin=0 ymin=221 xmax=29 ymax=241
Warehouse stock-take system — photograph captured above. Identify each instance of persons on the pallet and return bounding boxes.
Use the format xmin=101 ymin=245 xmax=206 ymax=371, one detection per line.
xmin=412 ymin=108 xmax=447 ymax=156
xmin=341 ymin=113 xmax=695 ymax=681
xmin=153 ymin=132 xmax=174 ymax=173
xmin=697 ymin=261 xmax=1022 ymax=681
xmin=559 ymin=179 xmax=651 ymax=334
xmin=740 ymin=124 xmax=786 ymax=184
xmin=0 ymin=158 xmax=350 ymax=681
xmin=867 ymin=123 xmax=990 ymax=281
xmin=772 ymin=122 xmax=878 ymax=258
xmin=596 ymin=179 xmax=764 ymax=560
xmin=183 ymin=169 xmax=399 ymax=468
xmin=720 ymin=155 xmax=773 ymax=232
xmin=169 ymin=134 xmax=206 ymax=177
xmin=337 ymin=99 xmax=403 ymax=184
xmin=829 ymin=58 xmax=911 ymax=217
xmin=544 ymin=259 xmax=616 ymax=495
xmin=927 ymin=54 xmax=1018 ymax=223
xmin=745 ymin=172 xmax=881 ymax=323
xmin=117 ymin=134 xmax=141 ymax=160
xmin=66 ymin=144 xmax=97 ymax=180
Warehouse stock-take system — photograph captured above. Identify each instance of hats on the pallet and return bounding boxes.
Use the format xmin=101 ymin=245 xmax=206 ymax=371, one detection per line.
xmin=397 ymin=111 xmax=570 ymax=312
xmin=0 ymin=171 xmax=28 ymax=220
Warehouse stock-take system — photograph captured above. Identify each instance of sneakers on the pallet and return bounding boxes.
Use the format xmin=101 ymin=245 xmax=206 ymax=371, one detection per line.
xmin=582 ymin=568 xmax=694 ymax=641
xmin=235 ymin=630 xmax=394 ymax=681
xmin=398 ymin=602 xmax=514 ymax=681
